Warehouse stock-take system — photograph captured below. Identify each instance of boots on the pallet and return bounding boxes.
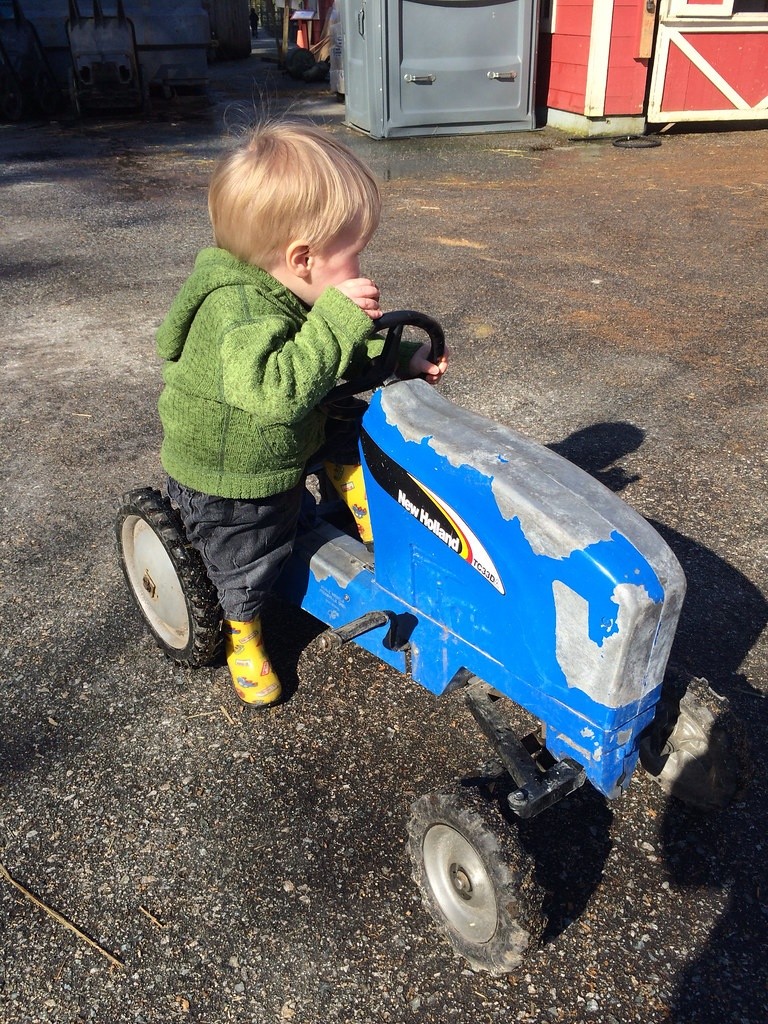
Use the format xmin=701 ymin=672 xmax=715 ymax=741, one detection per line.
xmin=322 ymin=448 xmax=374 ymax=553
xmin=225 ymin=617 xmax=281 ymax=707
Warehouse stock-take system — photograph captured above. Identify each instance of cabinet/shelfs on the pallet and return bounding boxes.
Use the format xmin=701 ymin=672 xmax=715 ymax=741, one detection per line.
xmin=338 ymin=0 xmax=540 ymax=141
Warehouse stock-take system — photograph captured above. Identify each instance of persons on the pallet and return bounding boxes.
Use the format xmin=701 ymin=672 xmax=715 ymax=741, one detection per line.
xmin=249 ymin=8 xmax=259 ymax=40
xmin=154 ymin=121 xmax=451 ymax=707
